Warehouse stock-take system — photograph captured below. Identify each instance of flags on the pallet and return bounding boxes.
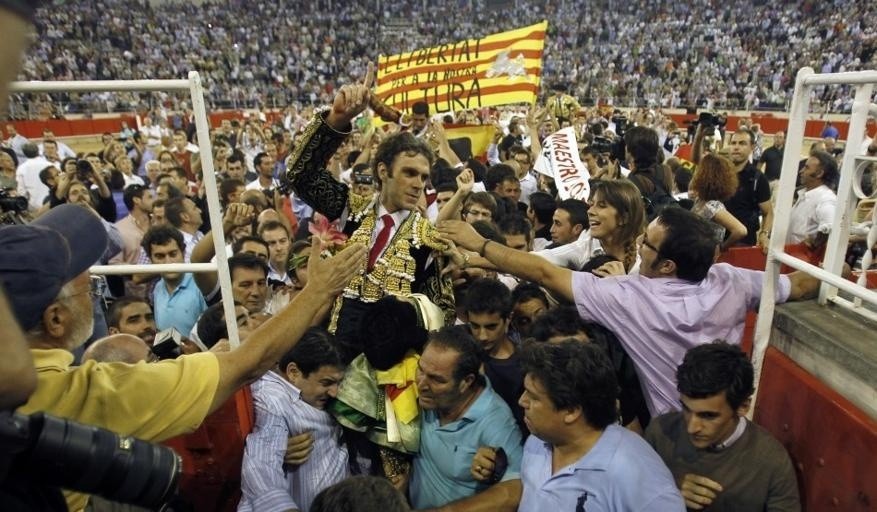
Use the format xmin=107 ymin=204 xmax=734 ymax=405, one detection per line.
xmin=372 ymin=18 xmax=551 ymax=111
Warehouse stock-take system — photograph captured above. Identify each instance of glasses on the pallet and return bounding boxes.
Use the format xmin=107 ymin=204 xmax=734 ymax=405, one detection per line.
xmin=56 ymin=275 xmax=106 ymax=300
xmin=130 ymin=184 xmax=141 ymax=196
xmin=516 ymin=160 xmax=529 ymax=165
xmin=642 ymin=232 xmax=660 ymax=256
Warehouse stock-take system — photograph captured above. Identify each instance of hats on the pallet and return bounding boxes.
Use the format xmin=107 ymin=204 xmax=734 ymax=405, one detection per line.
xmin=0 ymin=203 xmax=109 ymax=331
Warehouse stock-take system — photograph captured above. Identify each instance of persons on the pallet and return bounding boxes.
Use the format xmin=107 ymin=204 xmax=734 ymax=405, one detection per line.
xmin=347 ymin=0 xmax=543 ymax=56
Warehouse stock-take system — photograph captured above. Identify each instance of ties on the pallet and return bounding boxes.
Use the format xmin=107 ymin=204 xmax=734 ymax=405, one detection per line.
xmin=367 ymin=215 xmax=395 ymax=273
